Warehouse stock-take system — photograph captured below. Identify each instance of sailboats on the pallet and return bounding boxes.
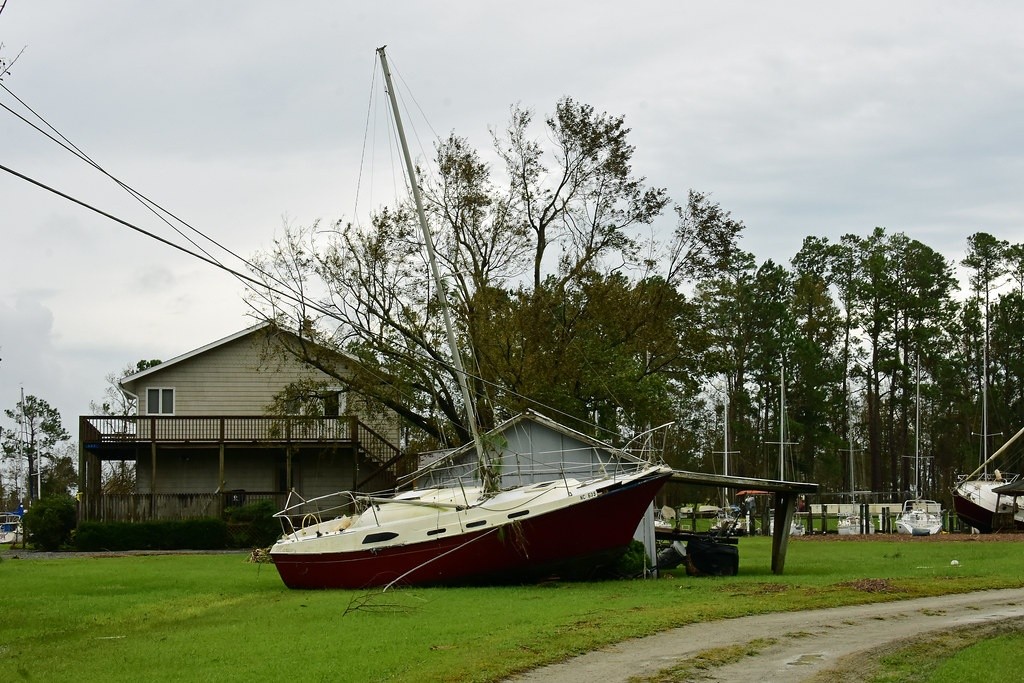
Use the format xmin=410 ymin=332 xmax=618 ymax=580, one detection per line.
xmin=769 ymin=365 xmax=806 ymax=536
xmin=950 ymin=427 xmax=1024 ymax=533
xmin=710 ymin=371 xmax=742 ymax=530
xmin=893 ymin=354 xmax=943 ymax=535
xmin=836 ymin=382 xmax=874 ymax=534
xmin=266 ymin=43 xmax=676 ymax=591
